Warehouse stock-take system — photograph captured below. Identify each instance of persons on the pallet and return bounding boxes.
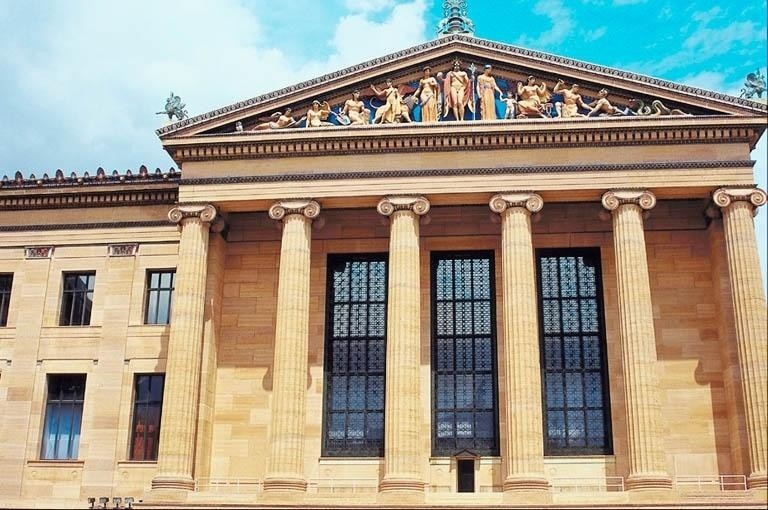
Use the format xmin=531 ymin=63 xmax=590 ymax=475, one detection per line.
xmin=438 ymin=60 xmax=475 ymax=121
xmin=588 ymin=90 xmax=637 ymax=118
xmin=305 ymin=101 xmax=334 ymax=128
xmin=476 ymin=65 xmax=503 ymax=120
xmin=414 ymin=66 xmax=442 ymax=122
xmin=342 ymin=90 xmax=371 ymax=126
xmin=516 ymin=76 xmax=547 ymax=119
xmin=553 ymin=79 xmax=593 ymax=117
xmin=370 ymin=79 xmax=404 ymax=123
xmin=499 ymin=91 xmax=518 ymax=119
xmin=251 ymin=108 xmax=307 ymax=129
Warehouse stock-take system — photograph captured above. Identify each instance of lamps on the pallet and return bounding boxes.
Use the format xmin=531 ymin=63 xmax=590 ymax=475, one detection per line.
xmin=88 ymin=497 xmax=135 ymax=510
